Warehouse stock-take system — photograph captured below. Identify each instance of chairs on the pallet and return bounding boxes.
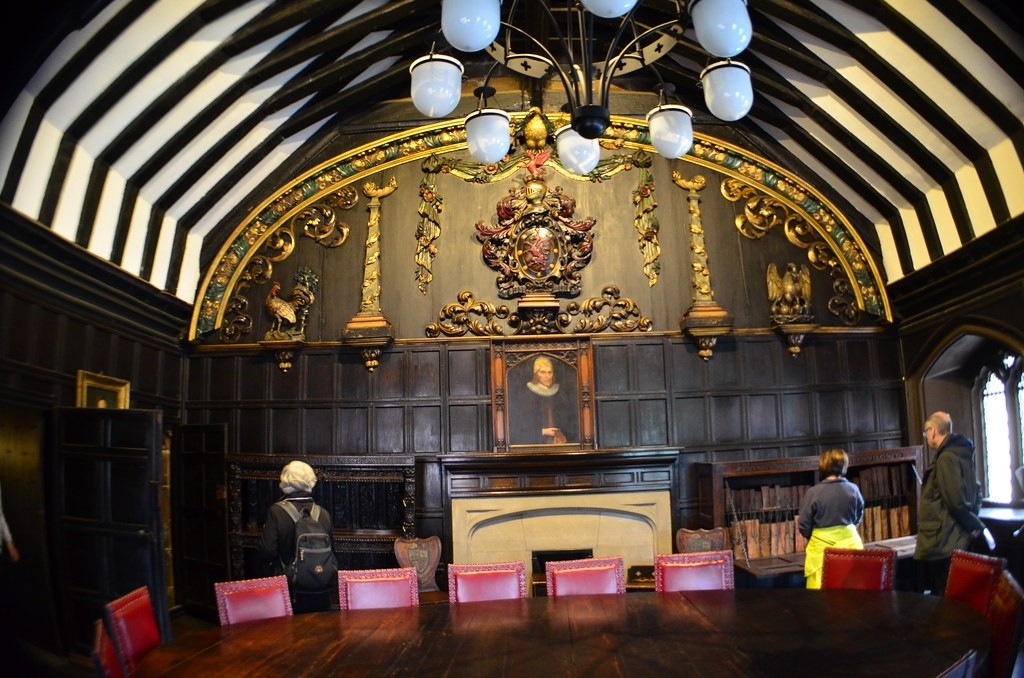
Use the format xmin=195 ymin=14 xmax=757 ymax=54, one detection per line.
xmin=654 ymin=550 xmax=735 ymax=591
xmin=945 ymin=549 xmax=1008 ymax=619
xmin=337 ymin=568 xmax=421 ymax=610
xmin=988 ymin=570 xmax=1024 ymax=678
xmin=105 ymin=586 xmax=163 ymax=666
xmin=448 ymin=563 xmax=528 ymax=604
xmin=545 ymin=558 xmax=627 ymax=595
xmin=214 ymin=576 xmax=294 ymax=626
xmin=822 ymin=548 xmax=897 ymax=589
xmin=93 ymin=618 xmax=125 ymax=678
xmin=394 ymin=536 xmax=448 ymax=605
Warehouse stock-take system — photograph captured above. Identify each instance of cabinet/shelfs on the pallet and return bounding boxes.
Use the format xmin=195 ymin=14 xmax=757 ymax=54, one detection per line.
xmin=694 ymin=445 xmax=924 ymax=580
xmin=43 ymin=405 xmax=173 ymax=650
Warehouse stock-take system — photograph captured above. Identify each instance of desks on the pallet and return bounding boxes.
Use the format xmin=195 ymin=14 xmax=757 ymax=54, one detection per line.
xmin=139 ymin=588 xmax=988 ymax=678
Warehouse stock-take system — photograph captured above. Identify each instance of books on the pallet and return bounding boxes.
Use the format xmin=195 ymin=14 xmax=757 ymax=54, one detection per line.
xmin=724 ymin=484 xmax=811 ymax=512
xmin=856 ymin=505 xmax=912 ymax=544
xmin=854 ymin=463 xmax=912 ymax=500
xmin=730 ymin=515 xmax=808 ymax=560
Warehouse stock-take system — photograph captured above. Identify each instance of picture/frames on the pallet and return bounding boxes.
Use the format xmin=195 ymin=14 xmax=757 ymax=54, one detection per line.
xmin=77 ymin=370 xmax=131 ymax=410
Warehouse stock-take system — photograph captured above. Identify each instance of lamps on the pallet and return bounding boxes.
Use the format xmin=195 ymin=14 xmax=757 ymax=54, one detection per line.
xmin=410 ymin=0 xmax=756 ymax=176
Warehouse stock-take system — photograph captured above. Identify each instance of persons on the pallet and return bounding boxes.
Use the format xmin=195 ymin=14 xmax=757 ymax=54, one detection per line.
xmin=912 ymin=411 xmax=987 ymax=597
xmin=0 ymin=507 xmax=20 ymax=562
xmin=797 ymin=449 xmax=865 ymax=589
xmin=255 ymin=461 xmax=337 ymax=615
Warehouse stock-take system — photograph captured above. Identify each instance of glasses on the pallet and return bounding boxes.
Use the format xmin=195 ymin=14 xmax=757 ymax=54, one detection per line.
xmin=923 ymin=427 xmax=934 ymax=437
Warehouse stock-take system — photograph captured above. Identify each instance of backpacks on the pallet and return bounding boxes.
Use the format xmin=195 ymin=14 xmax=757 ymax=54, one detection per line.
xmin=275 ymin=500 xmax=341 ymax=600
